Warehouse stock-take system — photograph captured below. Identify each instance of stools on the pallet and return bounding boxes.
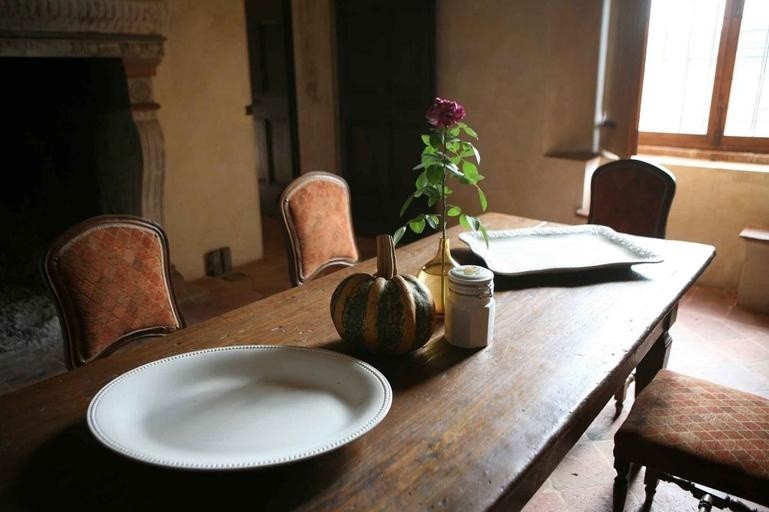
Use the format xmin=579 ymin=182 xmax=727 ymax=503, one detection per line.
xmin=736 ymin=223 xmax=769 ymax=312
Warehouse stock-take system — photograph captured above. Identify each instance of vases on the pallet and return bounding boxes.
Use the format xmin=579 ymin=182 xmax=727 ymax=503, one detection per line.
xmin=418 ymin=236 xmax=461 ymax=319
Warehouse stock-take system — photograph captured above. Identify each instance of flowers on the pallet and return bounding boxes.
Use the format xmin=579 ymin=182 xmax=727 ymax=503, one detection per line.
xmin=393 ymin=97 xmax=489 ymax=312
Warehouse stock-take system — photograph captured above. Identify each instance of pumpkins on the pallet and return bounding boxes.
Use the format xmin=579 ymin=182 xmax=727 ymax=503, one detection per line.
xmin=329 ymin=233 xmax=436 ymax=362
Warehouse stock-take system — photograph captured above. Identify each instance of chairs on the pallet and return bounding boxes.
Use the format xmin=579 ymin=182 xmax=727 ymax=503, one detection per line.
xmin=38 ymin=213 xmax=187 ymax=372
xmin=586 ymin=159 xmax=676 ymax=420
xmin=278 ymin=170 xmax=360 ymax=287
xmin=613 ymin=368 xmax=769 ymax=512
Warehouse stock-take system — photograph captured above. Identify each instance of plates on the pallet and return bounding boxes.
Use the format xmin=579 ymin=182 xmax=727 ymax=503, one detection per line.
xmin=457 ymin=224 xmax=664 ymax=277
xmin=85 ymin=343 xmax=392 ymax=475
